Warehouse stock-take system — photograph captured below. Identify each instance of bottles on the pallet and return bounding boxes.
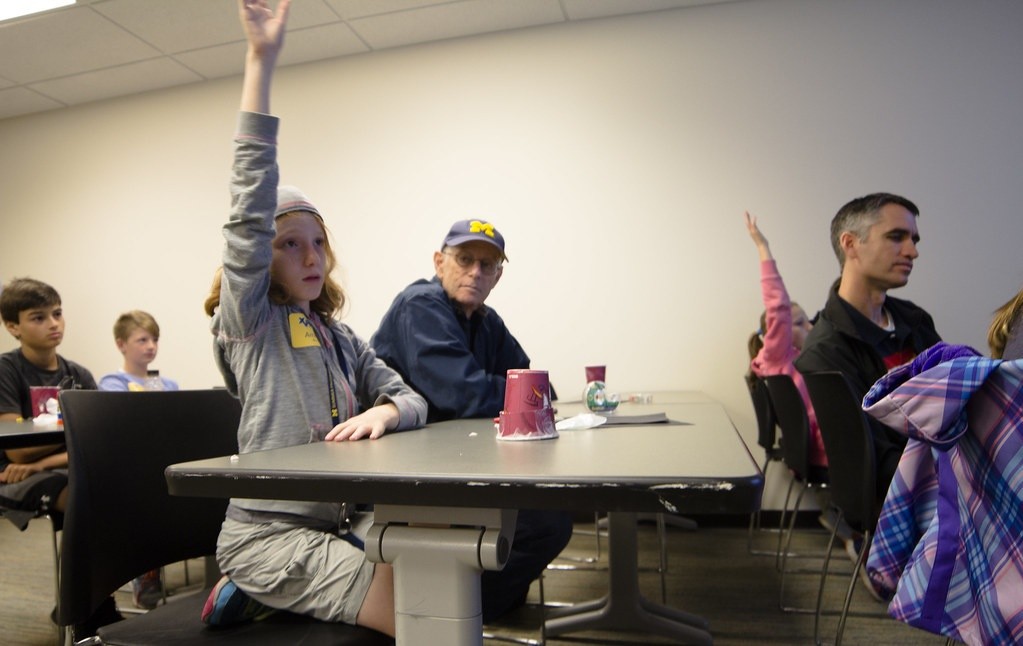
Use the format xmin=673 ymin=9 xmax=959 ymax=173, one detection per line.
xmin=145 ymin=369 xmax=163 ymax=391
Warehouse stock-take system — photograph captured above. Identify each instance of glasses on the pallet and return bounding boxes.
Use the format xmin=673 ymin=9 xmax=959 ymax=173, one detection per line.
xmin=442 ymin=250 xmax=502 ymax=274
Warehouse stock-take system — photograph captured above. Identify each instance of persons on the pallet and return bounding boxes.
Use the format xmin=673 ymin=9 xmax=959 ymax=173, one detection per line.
xmin=0 ymin=277 xmax=127 ymax=646
xmin=986 ymin=289 xmax=1023 ymax=361
xmin=206 ymin=0 xmax=427 ymax=639
xmin=742 ymin=210 xmax=827 ymax=466
xmin=99 ymin=311 xmax=181 ymax=610
xmin=369 ymin=220 xmax=573 ymax=629
xmin=796 ymin=192 xmax=947 ymax=527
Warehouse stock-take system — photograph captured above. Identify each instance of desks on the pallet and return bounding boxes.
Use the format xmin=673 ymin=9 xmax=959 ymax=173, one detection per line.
xmin=0 ymin=418 xmax=66 ymax=449
xmin=166 ymin=397 xmax=764 ymax=646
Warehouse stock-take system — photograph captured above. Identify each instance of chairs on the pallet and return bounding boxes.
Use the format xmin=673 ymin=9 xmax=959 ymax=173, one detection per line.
xmin=810 ymin=371 xmax=954 ymax=645
xmin=738 ymin=376 xmax=850 ymax=560
xmin=765 ymin=374 xmax=897 ymax=621
xmin=49 ymin=387 xmax=394 ymax=645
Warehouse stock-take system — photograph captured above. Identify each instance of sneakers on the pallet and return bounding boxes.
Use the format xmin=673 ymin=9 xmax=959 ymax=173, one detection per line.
xmin=130 ymin=566 xmax=163 ymax=609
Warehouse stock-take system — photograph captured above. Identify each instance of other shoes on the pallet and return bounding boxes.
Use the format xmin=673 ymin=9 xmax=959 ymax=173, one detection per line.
xmin=481 ymin=596 xmax=541 ymax=630
xmin=819 ymin=507 xmax=852 ymax=542
xmin=75 ymin=595 xmax=124 ymax=642
xmin=846 ymin=537 xmax=888 ymax=603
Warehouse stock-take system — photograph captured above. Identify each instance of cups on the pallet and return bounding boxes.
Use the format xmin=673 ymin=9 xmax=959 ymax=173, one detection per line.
xmin=496 ymin=367 xmax=561 ymax=440
xmin=30 ymin=385 xmax=60 ymax=418
xmin=582 ymin=365 xmax=606 ymax=383
xmin=583 ymin=380 xmax=620 ymax=415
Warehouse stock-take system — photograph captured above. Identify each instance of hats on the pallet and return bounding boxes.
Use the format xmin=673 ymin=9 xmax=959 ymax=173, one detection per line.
xmin=271 ymin=184 xmax=324 ymax=220
xmin=439 ymin=218 xmax=509 ymax=264
